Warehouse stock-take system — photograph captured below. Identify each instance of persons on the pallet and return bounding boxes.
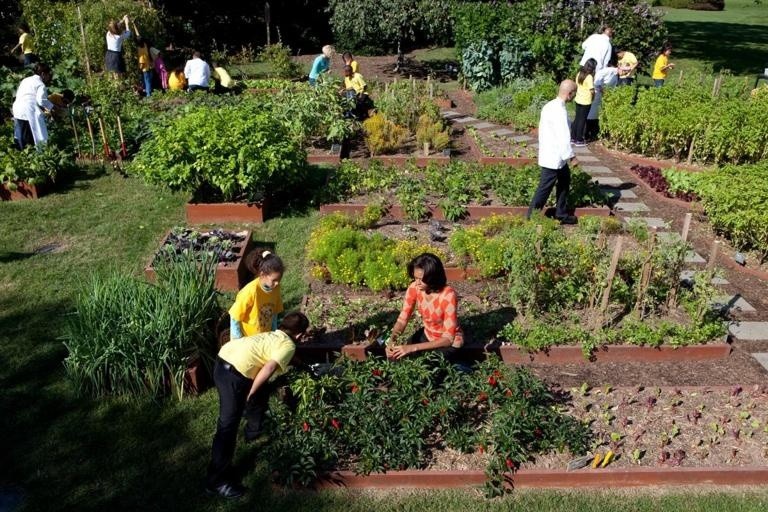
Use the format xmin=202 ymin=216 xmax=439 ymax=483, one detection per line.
xmin=9 ymin=21 xmax=39 ymax=65
xmin=386 ymin=253 xmax=465 ymax=371
xmin=102 ymin=14 xmax=233 ymax=95
xmin=571 ymin=57 xmax=598 ymax=146
xmin=48 ymin=89 xmax=77 ymax=111
xmin=581 ymin=28 xmax=613 ymax=71
xmin=615 ymin=48 xmax=638 ymax=86
xmin=205 ymin=313 xmax=309 ymax=498
xmin=651 ymin=45 xmax=673 ymax=85
xmin=309 ymin=44 xmax=336 ymax=85
xmin=12 ymin=61 xmax=57 ymax=151
xmin=338 ymin=65 xmax=366 ymax=104
xmin=585 ymin=60 xmax=618 ymax=141
xmin=525 ymin=78 xmax=580 ymax=223
xmin=343 ymin=52 xmax=359 ymax=71
xmin=229 ymin=249 xmax=283 ymax=339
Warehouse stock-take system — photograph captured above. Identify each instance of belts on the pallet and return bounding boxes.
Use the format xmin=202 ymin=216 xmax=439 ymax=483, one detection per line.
xmin=217 ymin=357 xmax=241 ymax=376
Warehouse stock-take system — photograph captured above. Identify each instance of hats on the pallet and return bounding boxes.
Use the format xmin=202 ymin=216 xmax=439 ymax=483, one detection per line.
xmin=322 ymin=45 xmax=336 ymax=59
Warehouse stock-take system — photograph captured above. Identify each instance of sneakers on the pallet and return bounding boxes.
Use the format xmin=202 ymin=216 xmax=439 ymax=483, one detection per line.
xmin=571 ymin=139 xmax=576 ymax=144
xmin=205 ymin=482 xmax=241 ymax=498
xmin=576 ymin=141 xmax=588 ymax=146
